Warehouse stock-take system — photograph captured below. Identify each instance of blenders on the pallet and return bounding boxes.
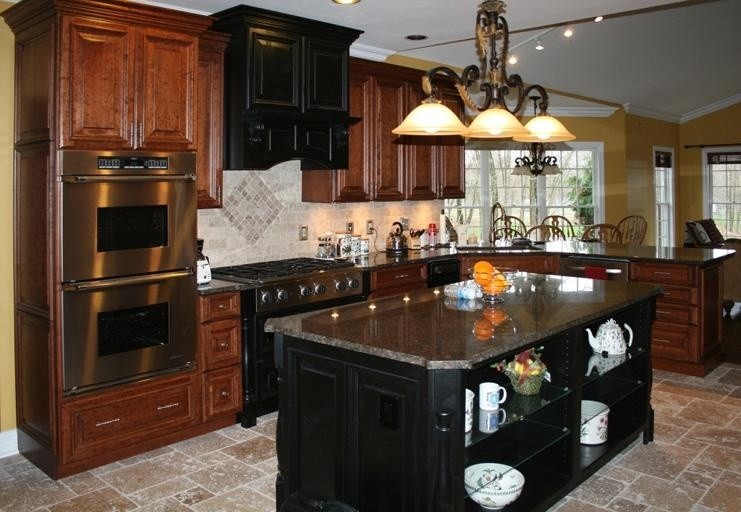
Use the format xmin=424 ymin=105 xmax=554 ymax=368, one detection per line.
xmin=196 ymin=239 xmax=214 ymax=285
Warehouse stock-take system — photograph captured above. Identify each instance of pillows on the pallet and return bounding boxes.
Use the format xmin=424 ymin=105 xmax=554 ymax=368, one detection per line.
xmin=686 ymin=219 xmax=723 ymax=246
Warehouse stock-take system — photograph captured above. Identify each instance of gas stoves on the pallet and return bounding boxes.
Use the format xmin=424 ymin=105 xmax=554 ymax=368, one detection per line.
xmin=212 ymin=257 xmax=355 ymax=279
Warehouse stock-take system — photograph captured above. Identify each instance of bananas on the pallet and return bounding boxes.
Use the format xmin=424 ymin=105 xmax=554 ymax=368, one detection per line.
xmin=493 ymin=266 xmax=506 ymax=285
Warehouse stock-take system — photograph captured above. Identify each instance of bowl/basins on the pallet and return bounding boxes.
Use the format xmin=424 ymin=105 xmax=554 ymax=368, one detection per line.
xmin=464 ymin=462 xmax=526 ymax=510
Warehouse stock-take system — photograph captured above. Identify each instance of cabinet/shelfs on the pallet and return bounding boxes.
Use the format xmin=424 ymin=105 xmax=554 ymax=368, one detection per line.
xmin=0 ymin=0 xmax=244 ymax=150
xmin=630 ymin=259 xmax=723 ymax=377
xmin=366 ymin=259 xmax=428 ymax=300
xmin=458 ymin=252 xmax=558 ymax=281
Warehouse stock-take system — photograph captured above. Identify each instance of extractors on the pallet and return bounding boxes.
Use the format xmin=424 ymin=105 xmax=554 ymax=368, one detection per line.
xmin=219 ymin=5 xmax=365 ymax=171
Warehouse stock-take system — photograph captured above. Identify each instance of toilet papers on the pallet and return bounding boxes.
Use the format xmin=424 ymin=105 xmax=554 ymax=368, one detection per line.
xmin=439 ymin=214 xmax=446 ymax=245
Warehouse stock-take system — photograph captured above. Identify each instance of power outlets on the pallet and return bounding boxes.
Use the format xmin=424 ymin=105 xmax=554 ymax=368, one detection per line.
xmin=299 ymin=224 xmax=308 ymax=240
xmin=367 ymin=220 xmax=373 ymax=234
xmin=401 ymin=218 xmax=409 ymax=230
xmin=346 ymin=220 xmax=354 ymax=235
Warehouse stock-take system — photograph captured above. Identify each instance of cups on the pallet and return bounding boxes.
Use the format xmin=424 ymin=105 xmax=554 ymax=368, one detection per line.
xmin=479 ymin=407 xmax=506 ymax=434
xmin=479 ymin=382 xmax=507 ymax=409
xmin=465 ymin=388 xmax=474 ymax=432
xmin=581 ymin=401 xmax=611 ymax=445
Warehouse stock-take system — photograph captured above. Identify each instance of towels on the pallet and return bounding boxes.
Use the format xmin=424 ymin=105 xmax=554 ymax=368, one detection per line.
xmin=560 ymin=257 xmax=577 ymax=276
xmin=584 ymin=264 xmax=609 ymax=281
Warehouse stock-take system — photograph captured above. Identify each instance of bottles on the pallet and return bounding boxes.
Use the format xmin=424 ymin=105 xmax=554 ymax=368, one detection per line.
xmin=318 ymin=244 xmax=325 ymax=257
xmin=326 ymin=243 xmax=333 ymax=256
xmin=350 ymin=236 xmax=361 ymax=256
xmin=361 ymin=239 xmax=369 ymax=254
xmin=443 ymin=286 xmax=483 ymax=300
xmin=428 ymin=223 xmax=437 ymax=248
xmin=439 ymin=209 xmax=446 ymax=244
xmin=336 ymin=233 xmax=351 ymax=257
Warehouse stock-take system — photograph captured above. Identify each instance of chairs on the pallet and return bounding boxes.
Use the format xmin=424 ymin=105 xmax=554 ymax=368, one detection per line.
xmin=491 ymin=202 xmax=648 ymax=247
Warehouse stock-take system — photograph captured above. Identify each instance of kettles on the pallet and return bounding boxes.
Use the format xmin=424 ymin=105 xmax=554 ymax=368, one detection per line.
xmin=386 ymin=221 xmax=408 ymax=257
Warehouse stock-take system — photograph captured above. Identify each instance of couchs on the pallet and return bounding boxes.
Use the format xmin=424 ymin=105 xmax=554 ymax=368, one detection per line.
xmin=683 ymin=239 xmax=741 ymax=321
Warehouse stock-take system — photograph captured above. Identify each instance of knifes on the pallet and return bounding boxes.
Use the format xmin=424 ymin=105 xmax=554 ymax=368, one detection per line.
xmin=410 ymin=227 xmax=425 ymax=237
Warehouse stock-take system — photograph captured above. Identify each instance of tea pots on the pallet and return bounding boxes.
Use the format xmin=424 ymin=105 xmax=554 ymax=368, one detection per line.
xmin=584 ymin=354 xmax=633 ymax=377
xmin=586 ymin=317 xmax=634 ymax=353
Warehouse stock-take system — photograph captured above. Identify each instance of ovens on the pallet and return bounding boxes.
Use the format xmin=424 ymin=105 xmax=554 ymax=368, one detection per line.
xmin=250 ymin=271 xmax=364 ymax=402
xmin=58 ymin=149 xmax=197 ymax=397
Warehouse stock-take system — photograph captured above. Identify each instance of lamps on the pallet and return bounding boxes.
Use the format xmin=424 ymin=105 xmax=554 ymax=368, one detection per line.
xmin=391 ymin=0 xmax=576 ymax=143
xmin=510 ymin=96 xmax=563 ymax=177
xmin=534 ymin=36 xmax=544 ymax=51
